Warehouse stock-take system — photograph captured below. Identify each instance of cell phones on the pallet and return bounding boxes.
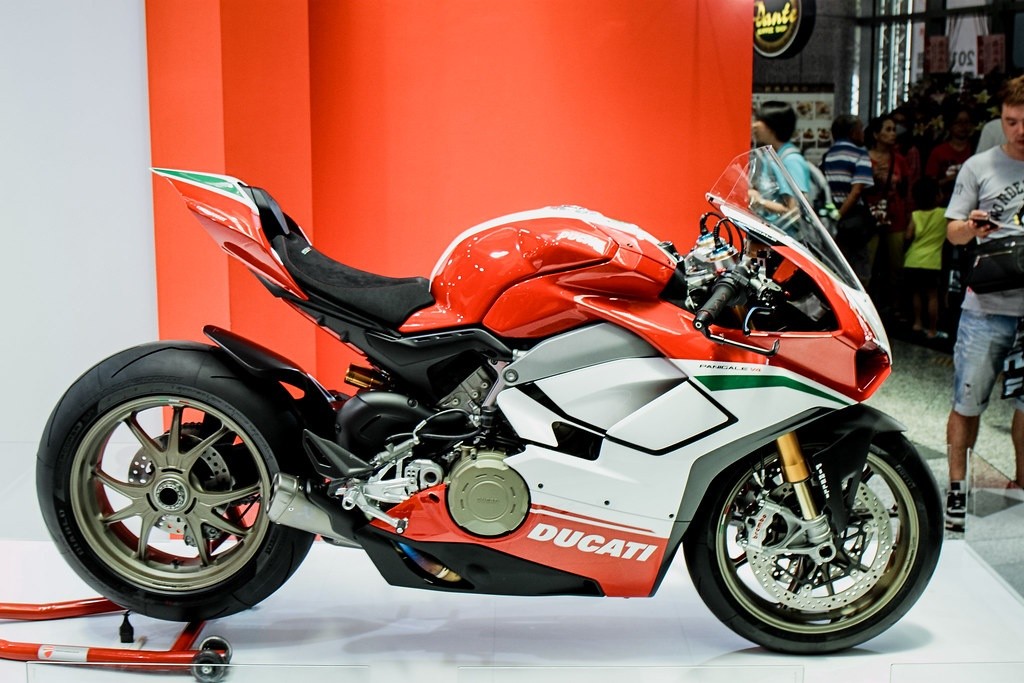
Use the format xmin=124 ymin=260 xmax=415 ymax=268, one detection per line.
xmin=969 ymin=216 xmax=999 ymax=229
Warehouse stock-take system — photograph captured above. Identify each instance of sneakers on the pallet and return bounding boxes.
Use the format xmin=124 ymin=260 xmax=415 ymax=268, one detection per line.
xmin=944 ymin=490 xmax=966 ymax=533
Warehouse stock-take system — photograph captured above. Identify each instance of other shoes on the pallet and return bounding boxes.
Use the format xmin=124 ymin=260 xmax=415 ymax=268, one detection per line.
xmin=1004 ymin=480 xmax=1023 ymax=505
xmin=879 ymin=307 xmax=907 ymax=323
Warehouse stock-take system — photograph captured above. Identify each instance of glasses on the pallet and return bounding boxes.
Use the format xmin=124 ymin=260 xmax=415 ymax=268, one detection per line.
xmin=952 ymin=119 xmax=969 ymax=125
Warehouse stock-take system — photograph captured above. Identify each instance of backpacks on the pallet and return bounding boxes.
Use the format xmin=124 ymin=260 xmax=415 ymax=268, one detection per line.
xmin=772 ymin=146 xmax=840 ymax=250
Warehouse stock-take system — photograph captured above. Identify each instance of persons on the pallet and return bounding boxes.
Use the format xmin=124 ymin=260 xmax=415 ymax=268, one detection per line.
xmin=943 ymin=76 xmax=1024 ymax=532
xmin=750 ymin=101 xmax=1009 ymax=350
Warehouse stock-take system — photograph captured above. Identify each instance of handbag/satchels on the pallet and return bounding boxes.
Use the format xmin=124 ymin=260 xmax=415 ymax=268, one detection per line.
xmin=835 ymin=203 xmax=877 ymax=256
xmin=956 ymin=234 xmax=1024 ymax=296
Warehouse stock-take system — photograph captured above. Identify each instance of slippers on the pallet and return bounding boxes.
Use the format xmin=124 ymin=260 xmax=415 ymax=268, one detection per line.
xmin=913 ymin=326 xmax=948 ymax=340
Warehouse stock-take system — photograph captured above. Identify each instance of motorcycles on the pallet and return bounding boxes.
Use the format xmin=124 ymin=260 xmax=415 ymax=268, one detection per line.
xmin=35 ymin=145 xmax=943 ymax=656
